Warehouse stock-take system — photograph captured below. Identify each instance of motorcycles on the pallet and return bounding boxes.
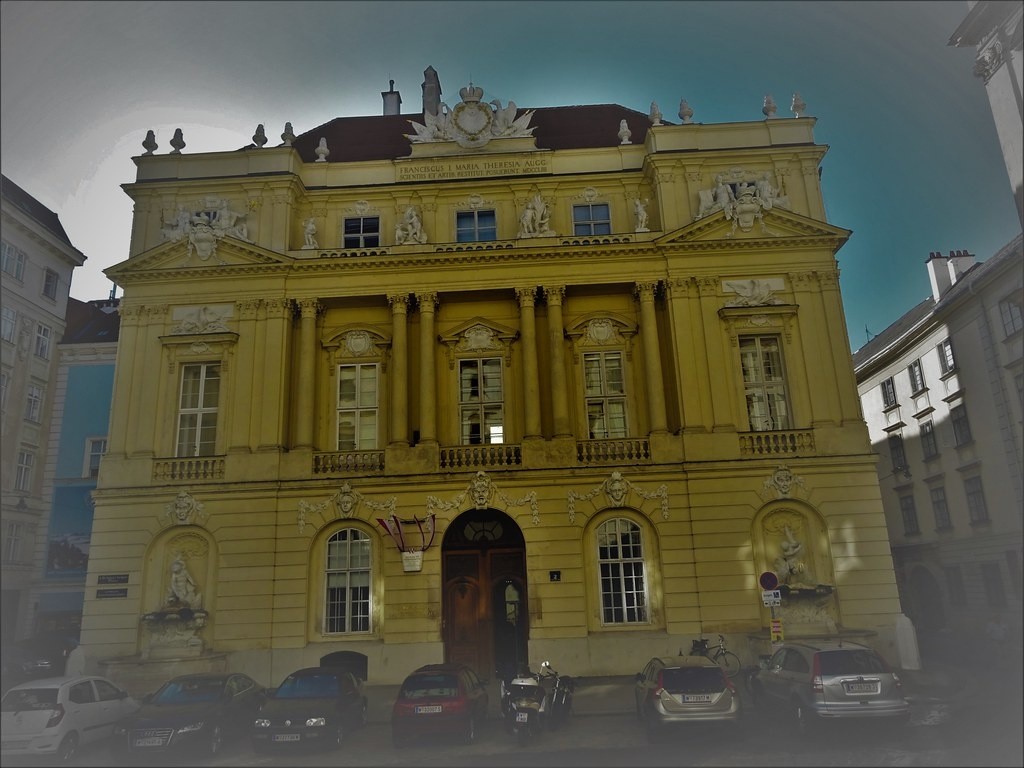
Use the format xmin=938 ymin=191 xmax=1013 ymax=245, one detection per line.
xmin=493 ymin=657 xmax=576 ymax=746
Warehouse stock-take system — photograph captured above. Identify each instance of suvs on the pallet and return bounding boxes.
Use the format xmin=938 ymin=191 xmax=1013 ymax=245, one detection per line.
xmin=393 ymin=661 xmax=489 ymax=748
xmin=633 ymin=655 xmax=741 ymax=736
xmin=760 ymin=639 xmax=910 ymax=737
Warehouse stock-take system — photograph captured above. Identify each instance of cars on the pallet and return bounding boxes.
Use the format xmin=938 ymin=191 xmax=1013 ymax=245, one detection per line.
xmin=249 ymin=650 xmax=368 ymax=755
xmin=0 ymin=673 xmax=142 ymax=765
xmin=0 ymin=630 xmax=84 ymax=688
xmin=111 ymin=672 xmax=266 ymax=765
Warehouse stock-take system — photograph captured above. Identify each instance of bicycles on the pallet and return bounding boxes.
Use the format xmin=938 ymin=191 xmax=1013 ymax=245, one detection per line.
xmin=679 ymin=634 xmax=741 ymax=678
